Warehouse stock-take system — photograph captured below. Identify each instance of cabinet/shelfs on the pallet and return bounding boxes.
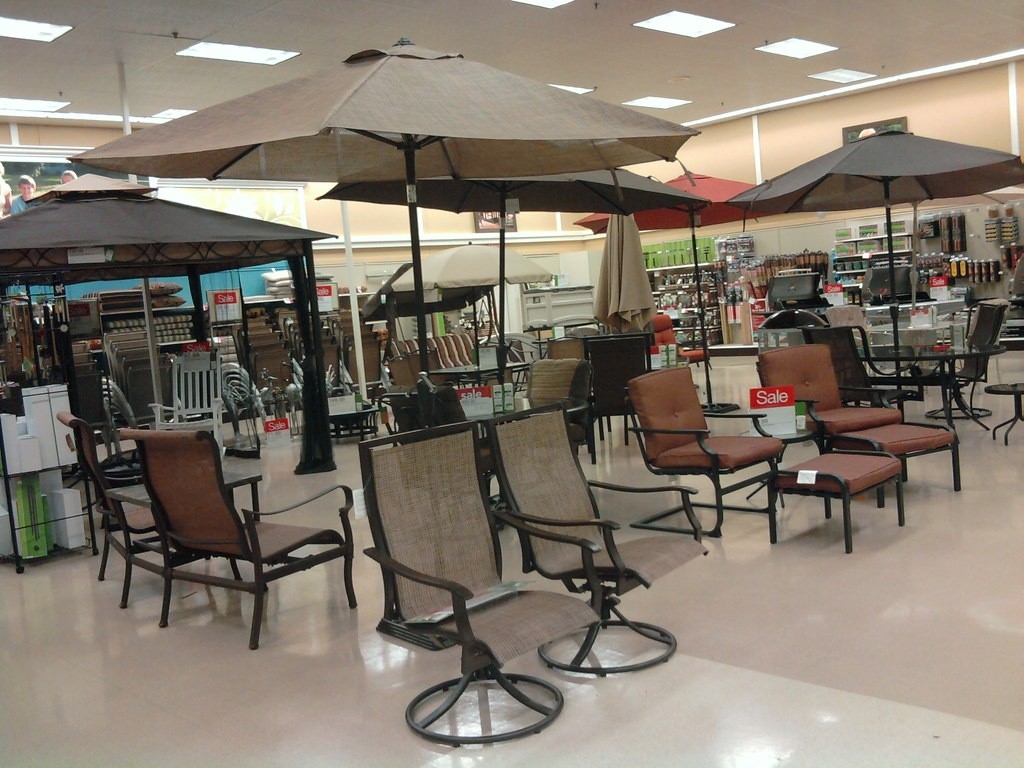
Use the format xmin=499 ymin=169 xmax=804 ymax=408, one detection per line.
xmin=644 ymin=261 xmax=723 ymax=346
xmin=831 ymin=232 xmax=913 ymax=288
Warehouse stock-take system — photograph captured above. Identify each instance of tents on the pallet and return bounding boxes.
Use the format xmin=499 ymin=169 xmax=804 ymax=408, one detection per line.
xmin=0 ymin=173 xmax=336 ymax=479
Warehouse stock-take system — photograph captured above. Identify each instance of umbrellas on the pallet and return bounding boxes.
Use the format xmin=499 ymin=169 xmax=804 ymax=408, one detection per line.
xmin=67 ymin=36 xmax=782 ymax=483
xmin=724 ymin=130 xmax=1024 ymax=483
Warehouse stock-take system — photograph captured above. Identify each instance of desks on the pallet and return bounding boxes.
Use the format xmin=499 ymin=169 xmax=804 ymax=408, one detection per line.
xmin=863 ymin=345 xmax=1006 ymax=432
xmin=426 ymin=364 xmax=529 ymax=386
xmin=461 ymin=396 xmax=590 ymax=524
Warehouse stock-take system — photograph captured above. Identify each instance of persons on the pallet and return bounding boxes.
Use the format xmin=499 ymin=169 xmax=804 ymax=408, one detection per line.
xmin=60 ymin=169 xmax=78 ymax=186
xmin=11 ymin=175 xmax=44 ymax=216
xmin=0 ymin=161 xmax=12 ymax=221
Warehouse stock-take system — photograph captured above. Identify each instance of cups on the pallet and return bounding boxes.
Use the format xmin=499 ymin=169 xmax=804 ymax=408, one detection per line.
xmin=796 ymin=415 xmax=806 ymax=430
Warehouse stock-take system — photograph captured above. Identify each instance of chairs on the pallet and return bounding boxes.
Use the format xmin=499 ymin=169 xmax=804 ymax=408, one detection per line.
xmin=57 ymin=301 xmax=1009 ymax=742
